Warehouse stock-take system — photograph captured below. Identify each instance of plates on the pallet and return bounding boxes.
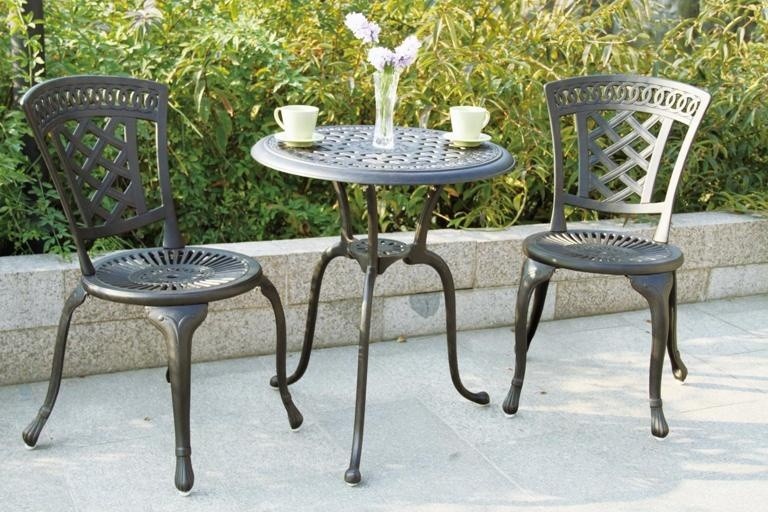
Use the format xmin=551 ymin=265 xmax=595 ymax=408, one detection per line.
xmin=272 ymin=131 xmax=326 ymax=148
xmin=442 ymin=132 xmax=492 ymax=148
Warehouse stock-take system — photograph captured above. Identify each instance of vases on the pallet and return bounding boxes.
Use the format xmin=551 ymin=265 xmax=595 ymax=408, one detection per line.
xmin=372 ymin=72 xmax=398 ymax=152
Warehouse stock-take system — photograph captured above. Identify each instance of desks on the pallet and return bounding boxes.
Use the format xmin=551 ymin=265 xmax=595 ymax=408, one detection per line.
xmin=248 ymin=126 xmax=517 ymax=487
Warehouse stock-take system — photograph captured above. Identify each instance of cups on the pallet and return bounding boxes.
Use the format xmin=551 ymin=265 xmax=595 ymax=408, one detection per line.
xmin=449 ymin=106 xmax=490 ymax=139
xmin=274 ymin=105 xmax=319 ymax=139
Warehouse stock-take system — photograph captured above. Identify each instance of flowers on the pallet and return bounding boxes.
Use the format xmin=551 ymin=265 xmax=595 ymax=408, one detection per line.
xmin=344 ymin=6 xmax=422 ymax=141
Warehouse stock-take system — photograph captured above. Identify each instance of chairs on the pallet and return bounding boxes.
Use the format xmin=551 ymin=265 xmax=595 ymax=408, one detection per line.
xmin=19 ymin=69 xmax=306 ymax=495
xmin=498 ymin=73 xmax=710 ymax=438
